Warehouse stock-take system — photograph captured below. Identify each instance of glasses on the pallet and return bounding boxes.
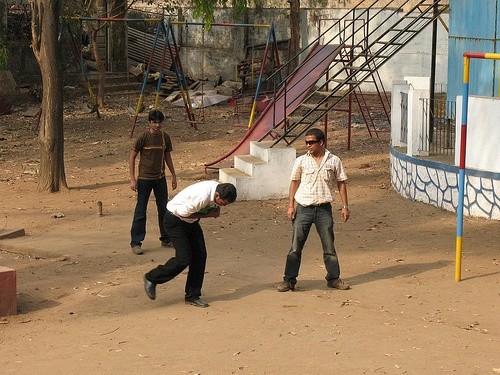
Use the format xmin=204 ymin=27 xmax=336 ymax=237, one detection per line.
xmin=152 ymin=120 xmax=161 ymax=124
xmin=305 ymin=141 xmax=320 ymax=145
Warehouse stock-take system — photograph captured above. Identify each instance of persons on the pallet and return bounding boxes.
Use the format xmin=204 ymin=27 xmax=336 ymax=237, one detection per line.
xmin=278 ymin=128 xmax=350 ymax=292
xmin=143 ymin=180 xmax=237 ymax=308
xmin=129 ymin=111 xmax=177 ymax=255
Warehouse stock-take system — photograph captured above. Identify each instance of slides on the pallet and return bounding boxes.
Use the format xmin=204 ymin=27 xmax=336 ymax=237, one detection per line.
xmin=204 ymin=43 xmax=343 ymax=175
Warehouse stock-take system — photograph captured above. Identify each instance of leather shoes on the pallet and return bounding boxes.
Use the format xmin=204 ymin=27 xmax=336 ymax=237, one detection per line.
xmin=185 ymin=298 xmax=208 ymax=307
xmin=145 ymin=275 xmax=155 ymax=300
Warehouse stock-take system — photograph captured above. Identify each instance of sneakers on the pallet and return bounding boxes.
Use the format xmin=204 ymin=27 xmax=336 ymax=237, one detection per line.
xmin=277 ymin=279 xmax=296 ymax=292
xmin=328 ymin=278 xmax=349 ymax=290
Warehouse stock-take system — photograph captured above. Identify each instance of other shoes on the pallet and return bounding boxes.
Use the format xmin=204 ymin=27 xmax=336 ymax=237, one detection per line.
xmin=132 ymin=245 xmax=144 ymax=255
xmin=162 ymin=241 xmax=173 ymax=247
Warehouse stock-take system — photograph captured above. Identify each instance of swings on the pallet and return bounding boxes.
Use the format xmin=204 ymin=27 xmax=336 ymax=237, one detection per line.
xmin=231 ymin=27 xmax=255 ymax=128
xmin=80 ymin=20 xmax=100 ymax=114
xmin=181 ymin=24 xmax=206 ymax=124
xmin=126 ymin=21 xmax=146 ymax=114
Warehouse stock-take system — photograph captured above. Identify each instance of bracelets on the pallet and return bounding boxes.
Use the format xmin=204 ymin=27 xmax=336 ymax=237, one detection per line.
xmin=342 ymin=205 xmax=348 ymax=208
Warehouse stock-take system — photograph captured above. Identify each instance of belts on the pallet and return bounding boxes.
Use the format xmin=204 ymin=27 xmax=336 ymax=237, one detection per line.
xmin=298 ymin=203 xmax=329 ymax=207
